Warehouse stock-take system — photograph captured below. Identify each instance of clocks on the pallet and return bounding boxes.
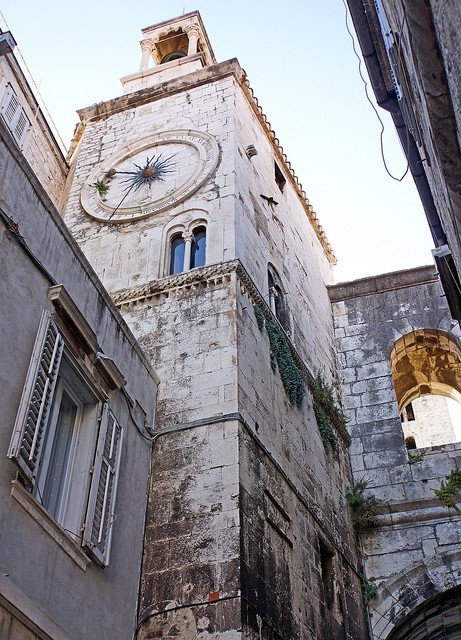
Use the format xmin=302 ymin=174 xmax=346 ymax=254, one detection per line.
xmin=78 ymin=128 xmax=220 ymax=225
